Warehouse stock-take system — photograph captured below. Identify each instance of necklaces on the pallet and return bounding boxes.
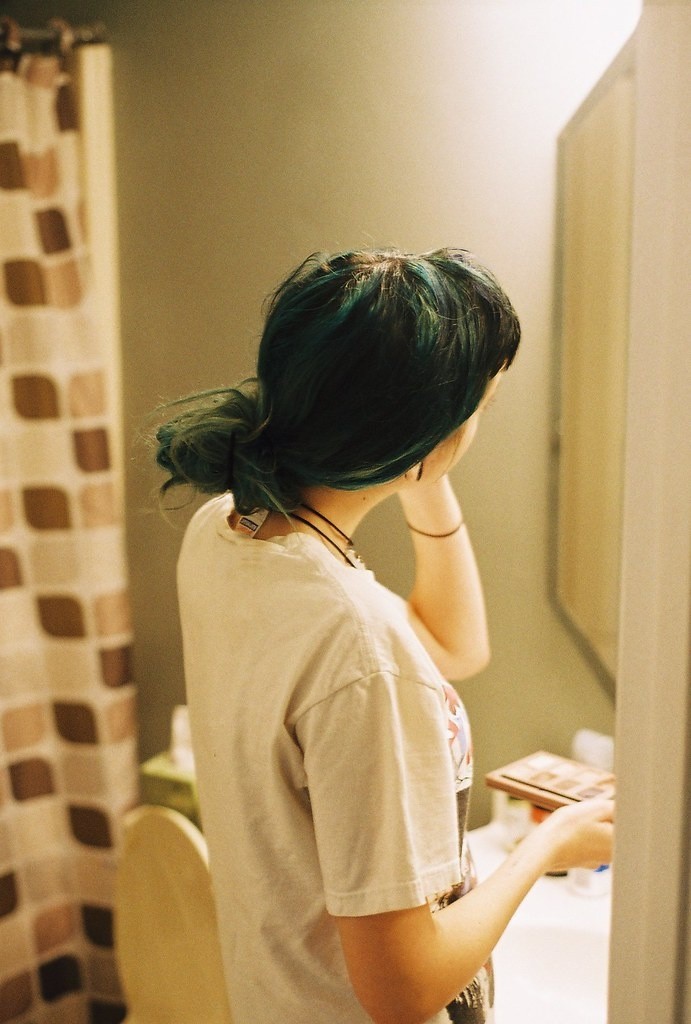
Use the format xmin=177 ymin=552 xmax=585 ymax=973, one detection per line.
xmin=285 ymin=497 xmax=357 ymax=569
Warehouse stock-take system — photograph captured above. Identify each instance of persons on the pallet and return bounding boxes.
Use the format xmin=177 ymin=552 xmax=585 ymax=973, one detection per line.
xmin=155 ymin=246 xmax=616 ymax=1024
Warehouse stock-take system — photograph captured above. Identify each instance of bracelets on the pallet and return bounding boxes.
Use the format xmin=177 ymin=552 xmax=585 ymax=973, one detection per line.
xmin=406 ymin=520 xmax=463 ymax=537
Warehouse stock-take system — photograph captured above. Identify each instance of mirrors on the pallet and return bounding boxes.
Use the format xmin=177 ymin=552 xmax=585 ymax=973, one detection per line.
xmin=539 ymin=21 xmax=636 ymax=708
